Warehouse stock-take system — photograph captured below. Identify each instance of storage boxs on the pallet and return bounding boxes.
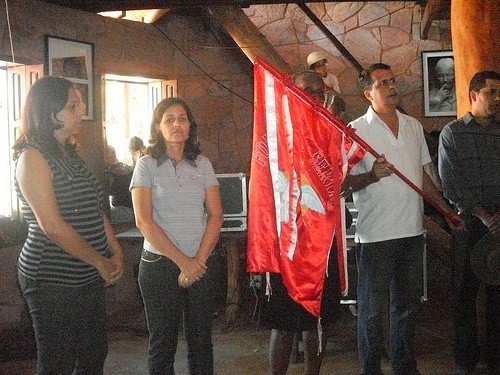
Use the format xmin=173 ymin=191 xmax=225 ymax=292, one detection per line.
xmin=339 ymin=202 xmax=428 ymax=305
xmin=214 ymin=172 xmax=250 ymax=232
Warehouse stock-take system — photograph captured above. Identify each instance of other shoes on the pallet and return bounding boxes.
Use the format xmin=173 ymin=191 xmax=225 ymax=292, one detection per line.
xmin=452 ymin=364 xmax=474 ymax=375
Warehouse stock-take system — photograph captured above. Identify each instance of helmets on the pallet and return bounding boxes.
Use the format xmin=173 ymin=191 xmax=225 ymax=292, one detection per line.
xmin=306 ymin=52 xmax=327 ymax=70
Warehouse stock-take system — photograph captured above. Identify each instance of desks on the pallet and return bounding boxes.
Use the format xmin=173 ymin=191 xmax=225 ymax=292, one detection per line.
xmin=114 ymin=227 xmax=247 ymax=335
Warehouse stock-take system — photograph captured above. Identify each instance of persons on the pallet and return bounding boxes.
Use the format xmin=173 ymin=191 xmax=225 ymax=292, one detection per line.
xmin=127 ymin=98 xmax=223 ymax=375
xmin=341 ymin=64 xmax=465 ymax=375
xmin=264 ymin=52 xmax=346 ymax=375
xmin=437 ymin=70 xmax=500 ymax=375
xmin=428 ymin=57 xmax=459 ymax=112
xmin=12 ymin=74 xmax=125 ymax=375
xmin=105 ymin=136 xmax=148 ymax=207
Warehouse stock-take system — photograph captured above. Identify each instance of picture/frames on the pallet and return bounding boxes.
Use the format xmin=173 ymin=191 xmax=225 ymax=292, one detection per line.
xmin=44 ymin=34 xmax=96 ymax=121
xmin=421 ymin=50 xmax=458 ymax=117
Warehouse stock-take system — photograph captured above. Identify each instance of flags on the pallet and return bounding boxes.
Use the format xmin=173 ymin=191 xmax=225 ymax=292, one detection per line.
xmin=246 ymin=57 xmax=369 ymax=317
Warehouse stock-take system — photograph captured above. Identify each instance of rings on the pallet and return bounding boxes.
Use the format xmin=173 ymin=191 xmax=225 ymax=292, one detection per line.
xmin=185 ymin=277 xmax=189 ymax=281
xmin=195 ymin=276 xmax=200 ymax=281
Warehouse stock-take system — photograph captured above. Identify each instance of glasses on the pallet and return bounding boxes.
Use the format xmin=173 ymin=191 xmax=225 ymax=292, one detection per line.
xmin=313 ymin=62 xmax=328 ymax=68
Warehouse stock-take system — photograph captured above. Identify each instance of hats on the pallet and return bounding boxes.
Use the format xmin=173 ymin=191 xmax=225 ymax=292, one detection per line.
xmin=470 ymin=231 xmax=500 ymax=285
